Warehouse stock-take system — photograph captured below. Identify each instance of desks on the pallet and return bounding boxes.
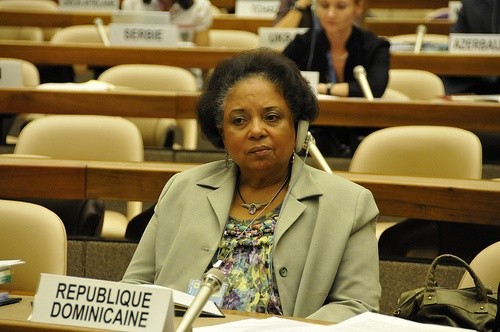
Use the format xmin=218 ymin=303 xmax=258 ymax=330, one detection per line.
xmin=0 ymin=157 xmax=500 ymax=227
xmin=0 ymin=39 xmax=500 ymax=77
xmin=0 ymin=288 xmax=337 ymax=332
xmin=0 ymin=87 xmax=500 ymax=134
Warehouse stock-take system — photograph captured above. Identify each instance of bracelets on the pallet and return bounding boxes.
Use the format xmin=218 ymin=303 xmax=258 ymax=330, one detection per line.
xmin=293 ymin=5 xmax=305 ymax=13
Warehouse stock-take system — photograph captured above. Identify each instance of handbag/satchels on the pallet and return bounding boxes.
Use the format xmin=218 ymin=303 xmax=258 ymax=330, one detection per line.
xmin=393 ymin=255 xmax=497 ymax=332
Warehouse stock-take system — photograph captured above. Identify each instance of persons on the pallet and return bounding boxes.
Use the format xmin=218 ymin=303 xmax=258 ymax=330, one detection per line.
xmin=441 ymin=0 xmax=500 ymax=165
xmin=273 ymin=0 xmax=325 ymax=30
xmin=281 ymin=0 xmax=391 ymax=159
xmin=121 ymin=47 xmax=380 ymax=323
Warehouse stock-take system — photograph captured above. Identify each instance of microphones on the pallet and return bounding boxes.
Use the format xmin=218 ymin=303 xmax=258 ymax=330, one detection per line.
xmin=303 ymin=131 xmax=333 ymax=175
xmin=176 ymin=267 xmax=225 ymax=332
xmin=414 ymin=25 xmax=426 ymax=52
xmin=354 ymin=65 xmax=374 ymax=99
xmin=93 ymin=17 xmax=110 ymax=45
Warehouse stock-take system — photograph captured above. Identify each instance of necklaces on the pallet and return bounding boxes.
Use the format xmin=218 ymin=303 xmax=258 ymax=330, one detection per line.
xmin=213 ymin=175 xmax=291 ymax=269
xmin=236 ymin=178 xmax=290 ymax=215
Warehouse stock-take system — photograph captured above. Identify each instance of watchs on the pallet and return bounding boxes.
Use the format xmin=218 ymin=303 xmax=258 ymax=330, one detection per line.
xmin=326 ymin=82 xmax=334 ymax=95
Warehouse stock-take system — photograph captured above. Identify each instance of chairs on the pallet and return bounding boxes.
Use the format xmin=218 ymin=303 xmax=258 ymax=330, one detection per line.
xmin=0 ymin=0 xmax=500 ymax=297
